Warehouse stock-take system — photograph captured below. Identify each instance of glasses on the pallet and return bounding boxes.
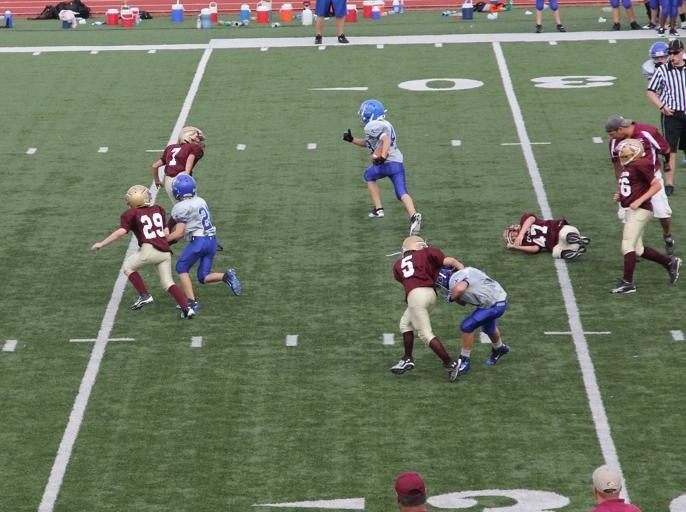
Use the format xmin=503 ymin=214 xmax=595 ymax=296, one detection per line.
xmin=668 ymin=51 xmax=681 ymax=55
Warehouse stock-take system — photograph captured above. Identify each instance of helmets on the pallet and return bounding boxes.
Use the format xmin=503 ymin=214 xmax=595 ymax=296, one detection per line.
xmin=437 ymin=266 xmax=455 ymax=290
xmin=172 ymin=174 xmax=196 ymax=200
xmin=359 ymin=100 xmax=384 ymax=123
xmin=402 ymin=235 xmax=428 ymax=254
xmin=651 ymin=42 xmax=669 ymax=57
xmin=503 ymin=225 xmax=521 ymax=244
xmin=179 ymin=126 xmax=205 ymax=145
xmin=616 ymin=139 xmax=644 ymax=166
xmin=126 ymin=184 xmax=152 ymax=208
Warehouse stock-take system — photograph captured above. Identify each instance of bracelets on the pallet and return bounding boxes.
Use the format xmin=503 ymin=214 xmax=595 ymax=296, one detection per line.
xmin=660 ymin=104 xmax=666 ymax=110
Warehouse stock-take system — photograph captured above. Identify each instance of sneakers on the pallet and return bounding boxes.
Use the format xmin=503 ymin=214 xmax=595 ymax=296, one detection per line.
xmin=561 ymin=245 xmax=586 ymax=260
xmin=449 ymin=358 xmax=461 ymax=383
xmin=176 ymin=302 xmax=199 ymax=311
xmin=659 ymin=27 xmax=664 ymax=37
xmin=613 ymin=23 xmax=620 ymax=31
xmin=669 ymin=29 xmax=679 ymax=36
xmin=130 ymin=293 xmax=153 ymax=310
xmin=566 ymin=232 xmax=590 ymax=245
xmin=459 ymin=355 xmax=470 ymax=374
xmin=180 ymin=308 xmax=196 ymax=319
xmin=630 ymin=21 xmax=642 ymax=30
xmin=338 ymin=32 xmax=349 ymax=43
xmin=486 ymin=344 xmax=510 ymax=366
xmin=663 ymin=233 xmax=674 ymax=255
xmin=665 ymin=185 xmax=673 ymax=195
xmin=315 ymin=35 xmax=322 ymax=44
xmin=557 ymin=24 xmax=565 ymax=32
xmin=609 ymin=280 xmax=636 ymax=294
xmin=536 ymin=24 xmax=544 ymax=33
xmin=225 ymin=268 xmax=240 ymax=296
xmin=642 ymin=22 xmax=656 ymax=30
xmin=666 ymin=255 xmax=680 ymax=284
xmin=391 ymin=358 xmax=415 ymax=375
xmin=410 ymin=212 xmax=421 ymax=235
xmin=369 ymin=208 xmax=384 ymax=217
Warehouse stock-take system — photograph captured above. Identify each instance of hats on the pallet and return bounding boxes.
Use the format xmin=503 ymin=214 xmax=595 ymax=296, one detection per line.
xmin=395 ymin=472 xmax=425 ymax=499
xmin=666 ymin=40 xmax=684 ymax=50
xmin=605 ymin=114 xmax=632 ymax=131
xmin=592 ymin=464 xmax=622 ymax=494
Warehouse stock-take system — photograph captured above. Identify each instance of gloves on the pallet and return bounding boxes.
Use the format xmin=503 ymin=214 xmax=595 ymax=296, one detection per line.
xmin=373 ymin=156 xmax=386 ymax=166
xmin=343 ymin=129 xmax=353 ymax=142
xmin=664 ymin=164 xmax=670 ymax=172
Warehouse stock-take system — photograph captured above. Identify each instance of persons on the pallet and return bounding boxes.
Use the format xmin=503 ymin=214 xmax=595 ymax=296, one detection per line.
xmin=390 ymin=234 xmax=464 ymax=381
xmin=647 ymin=38 xmax=686 ymax=195
xmin=150 ymin=127 xmax=223 ymax=253
xmin=535 ymin=0 xmax=567 ymax=33
xmin=642 ymin=42 xmax=685 ymax=82
xmin=588 ymin=464 xmax=640 ymax=512
xmin=315 ymin=0 xmax=351 ymax=44
xmin=609 ymin=138 xmax=682 ymax=295
xmin=167 ymin=172 xmax=242 ymax=310
xmin=611 ymin=1 xmax=686 ymax=37
xmin=342 ymin=98 xmax=423 ymax=235
xmin=395 ymin=470 xmax=430 ymax=512
xmin=604 ymin=116 xmax=676 ymax=264
xmin=89 ymin=186 xmax=195 ymax=320
xmin=433 ymin=262 xmax=511 ymax=374
xmin=504 ymin=213 xmax=591 ymax=260
